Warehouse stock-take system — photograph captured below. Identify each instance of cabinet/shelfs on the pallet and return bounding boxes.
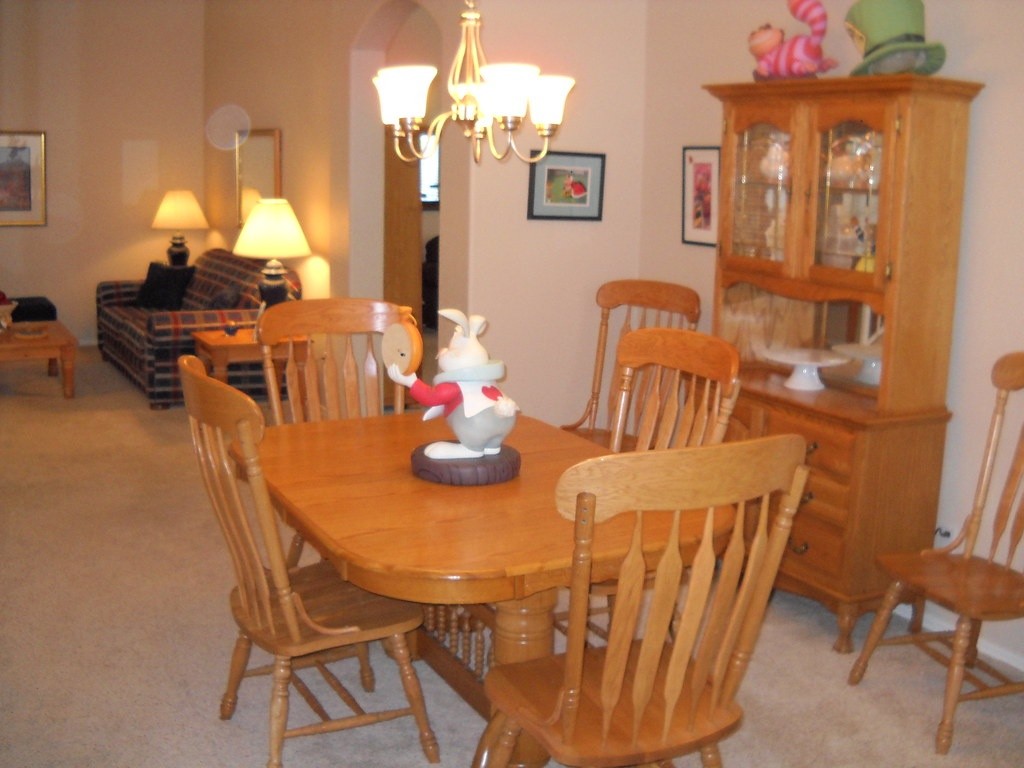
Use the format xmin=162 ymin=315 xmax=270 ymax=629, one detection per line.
xmin=703 ymin=81 xmax=986 ymax=655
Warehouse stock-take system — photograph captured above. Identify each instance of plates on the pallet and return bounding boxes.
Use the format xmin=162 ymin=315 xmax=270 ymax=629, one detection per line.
xmin=14 ymin=329 xmax=49 ymax=341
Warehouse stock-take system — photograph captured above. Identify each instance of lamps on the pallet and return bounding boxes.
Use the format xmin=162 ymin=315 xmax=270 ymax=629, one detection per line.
xmin=232 ymin=198 xmax=314 ymax=307
xmin=151 ymin=190 xmax=209 ymax=269
xmin=371 ymin=0 xmax=577 ymax=164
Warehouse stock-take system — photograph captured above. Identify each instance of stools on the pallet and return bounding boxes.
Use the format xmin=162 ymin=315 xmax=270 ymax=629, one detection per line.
xmin=10 ymin=297 xmax=57 ymax=323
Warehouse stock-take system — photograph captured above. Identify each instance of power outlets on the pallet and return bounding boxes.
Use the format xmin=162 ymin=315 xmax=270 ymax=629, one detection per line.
xmin=940 ymin=528 xmax=952 ymax=548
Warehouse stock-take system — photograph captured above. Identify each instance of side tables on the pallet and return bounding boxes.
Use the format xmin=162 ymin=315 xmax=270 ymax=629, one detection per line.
xmin=193 ymin=329 xmax=309 ymax=398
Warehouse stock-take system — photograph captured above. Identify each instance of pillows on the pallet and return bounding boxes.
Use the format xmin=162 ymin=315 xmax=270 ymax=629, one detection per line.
xmin=136 ymin=262 xmax=196 ymax=310
xmin=213 ymin=274 xmax=245 ymax=309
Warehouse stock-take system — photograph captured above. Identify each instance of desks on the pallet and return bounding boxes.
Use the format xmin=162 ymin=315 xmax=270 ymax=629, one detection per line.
xmin=228 ymin=409 xmax=735 ymax=767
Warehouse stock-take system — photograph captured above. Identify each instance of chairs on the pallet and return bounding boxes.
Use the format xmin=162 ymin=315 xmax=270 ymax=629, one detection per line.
xmin=177 ymin=353 xmax=442 ymax=768
xmin=847 ymin=351 xmax=1024 ymax=755
xmin=255 ymin=297 xmax=413 ymax=574
xmin=486 ymin=433 xmax=811 ymax=768
xmin=550 ymin=280 xmax=738 ymax=655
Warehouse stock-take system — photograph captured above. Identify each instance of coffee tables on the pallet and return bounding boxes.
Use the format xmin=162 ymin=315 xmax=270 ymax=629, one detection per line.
xmin=0 ymin=320 xmax=79 ymax=397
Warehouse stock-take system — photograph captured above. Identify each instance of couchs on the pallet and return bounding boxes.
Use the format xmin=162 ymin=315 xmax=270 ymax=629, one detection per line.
xmin=96 ymin=248 xmax=302 ymax=411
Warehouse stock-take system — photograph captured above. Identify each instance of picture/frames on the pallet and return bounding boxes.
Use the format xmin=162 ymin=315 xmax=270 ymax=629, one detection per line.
xmin=526 ymin=150 xmax=607 ymax=220
xmin=682 ymin=146 xmax=721 ymax=246
xmin=234 ymin=129 xmax=283 ymax=229
xmin=0 ymin=128 xmax=48 ymax=226
